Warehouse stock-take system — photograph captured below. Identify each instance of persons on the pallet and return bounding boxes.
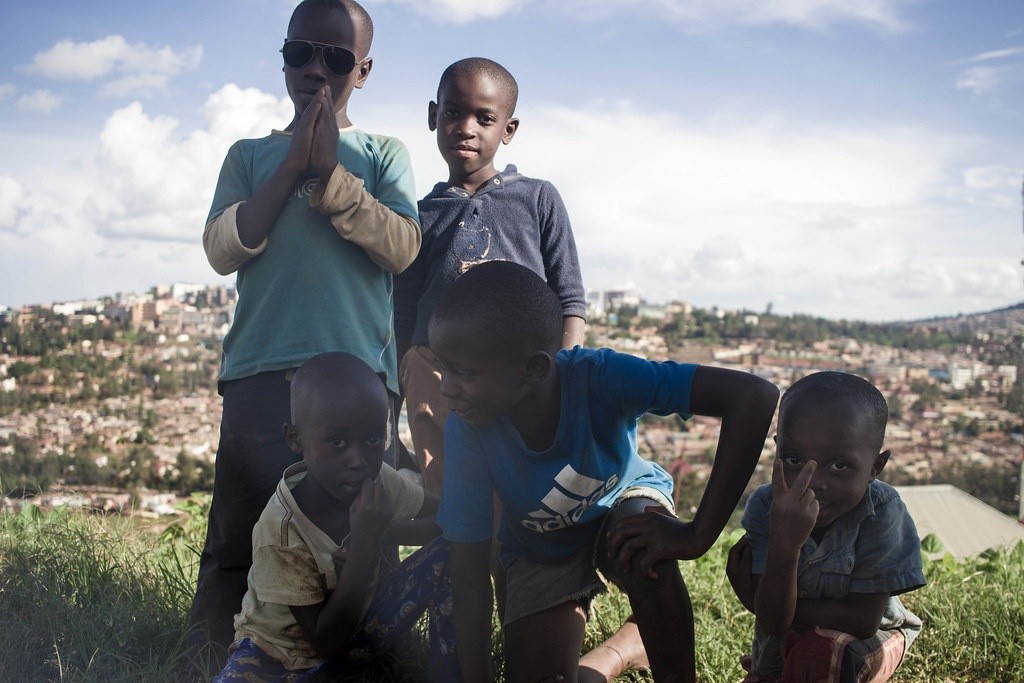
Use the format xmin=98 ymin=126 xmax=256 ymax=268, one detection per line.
xmin=427 ymin=260 xmax=780 ymax=683
xmin=726 ymin=371 xmax=927 ymax=683
xmin=213 ymin=352 xmax=462 ymax=683
xmin=178 ymin=1 xmax=422 ymax=683
xmin=394 ymin=58 xmax=587 ymax=628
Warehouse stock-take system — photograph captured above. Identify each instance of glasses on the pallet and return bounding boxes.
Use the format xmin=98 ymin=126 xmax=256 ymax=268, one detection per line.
xmin=278 ymin=39 xmax=370 ymax=76
